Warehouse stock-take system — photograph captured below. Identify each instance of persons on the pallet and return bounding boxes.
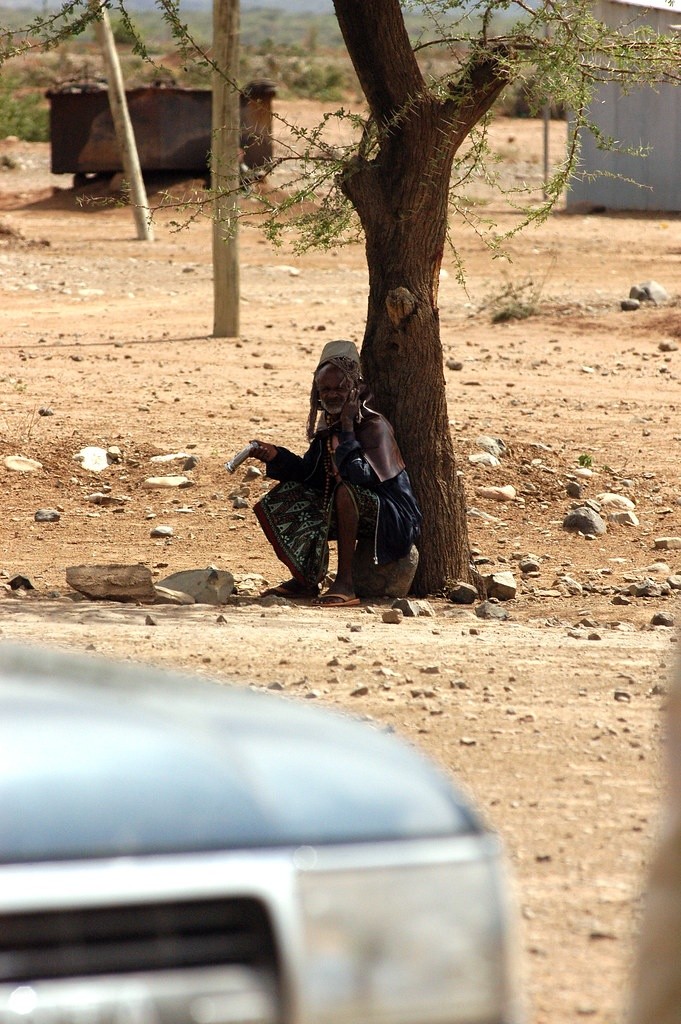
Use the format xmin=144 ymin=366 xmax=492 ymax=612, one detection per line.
xmin=248 ymin=339 xmax=423 ymax=608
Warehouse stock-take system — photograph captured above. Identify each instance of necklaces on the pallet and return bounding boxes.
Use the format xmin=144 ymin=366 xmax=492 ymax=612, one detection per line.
xmin=322 ymin=412 xmax=339 ymax=512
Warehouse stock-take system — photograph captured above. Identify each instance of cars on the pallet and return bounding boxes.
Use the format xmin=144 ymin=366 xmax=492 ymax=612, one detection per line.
xmin=0 ymin=642 xmax=518 ymax=1024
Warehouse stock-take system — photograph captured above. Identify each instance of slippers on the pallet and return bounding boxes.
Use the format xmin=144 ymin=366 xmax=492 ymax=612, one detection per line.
xmin=259 ymin=586 xmax=318 ymax=598
xmin=312 ymin=594 xmax=361 ymax=606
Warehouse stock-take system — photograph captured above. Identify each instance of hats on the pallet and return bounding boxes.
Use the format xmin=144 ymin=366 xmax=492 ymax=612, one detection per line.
xmin=307 ymin=340 xmax=365 ymax=410
